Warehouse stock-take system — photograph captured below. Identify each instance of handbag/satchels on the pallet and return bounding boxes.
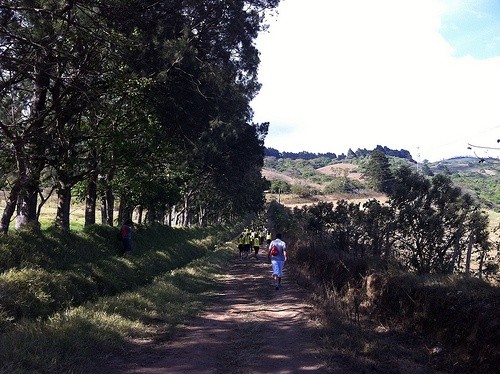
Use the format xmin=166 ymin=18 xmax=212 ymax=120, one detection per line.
xmin=117 ymin=234 xmax=122 ymax=241
xmin=268 ymin=245 xmax=278 ymax=256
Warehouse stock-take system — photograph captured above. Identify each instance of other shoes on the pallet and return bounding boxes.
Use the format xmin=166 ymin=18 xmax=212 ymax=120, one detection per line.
xmin=278 ymin=282 xmax=281 ymax=286
xmin=274 ymin=283 xmax=278 ymax=290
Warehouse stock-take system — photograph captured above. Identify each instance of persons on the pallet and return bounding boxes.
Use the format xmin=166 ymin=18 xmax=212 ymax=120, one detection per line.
xmin=121 ymin=219 xmax=134 ymax=250
xmin=238 ymin=224 xmax=272 ymax=258
xmin=268 ymin=233 xmax=287 ymax=290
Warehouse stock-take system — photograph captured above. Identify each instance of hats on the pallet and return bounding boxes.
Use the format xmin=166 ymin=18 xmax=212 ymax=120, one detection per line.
xmin=126 ymin=219 xmax=133 ymax=223
